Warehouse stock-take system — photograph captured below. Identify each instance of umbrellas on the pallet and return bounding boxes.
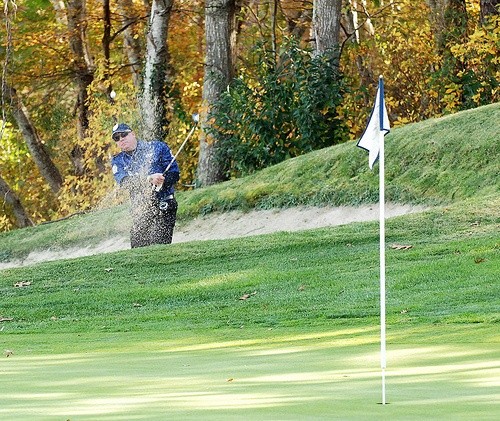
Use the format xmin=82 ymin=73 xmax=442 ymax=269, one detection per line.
xmin=354 ymin=75 xmax=391 ymax=171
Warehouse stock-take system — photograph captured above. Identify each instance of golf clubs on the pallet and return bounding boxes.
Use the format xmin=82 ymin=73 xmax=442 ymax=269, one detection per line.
xmin=153 ymin=113 xmax=199 ymax=195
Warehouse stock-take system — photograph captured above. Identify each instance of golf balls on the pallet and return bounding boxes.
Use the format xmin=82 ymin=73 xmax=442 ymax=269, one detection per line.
xmin=110 ymin=90 xmax=116 ymax=98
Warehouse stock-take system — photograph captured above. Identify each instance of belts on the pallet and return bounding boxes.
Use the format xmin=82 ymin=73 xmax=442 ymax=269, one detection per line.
xmin=159 ymin=193 xmax=175 ymax=201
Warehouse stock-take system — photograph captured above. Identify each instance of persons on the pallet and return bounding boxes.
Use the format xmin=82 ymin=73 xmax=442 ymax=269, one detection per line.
xmin=107 ymin=123 xmax=181 ymax=248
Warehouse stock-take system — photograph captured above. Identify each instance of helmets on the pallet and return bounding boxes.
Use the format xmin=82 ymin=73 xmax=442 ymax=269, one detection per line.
xmin=112 ymin=123 xmax=133 ymax=138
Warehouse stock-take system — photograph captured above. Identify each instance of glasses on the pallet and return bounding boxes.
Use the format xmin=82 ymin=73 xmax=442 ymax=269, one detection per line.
xmin=114 ymin=130 xmax=131 ymax=140
